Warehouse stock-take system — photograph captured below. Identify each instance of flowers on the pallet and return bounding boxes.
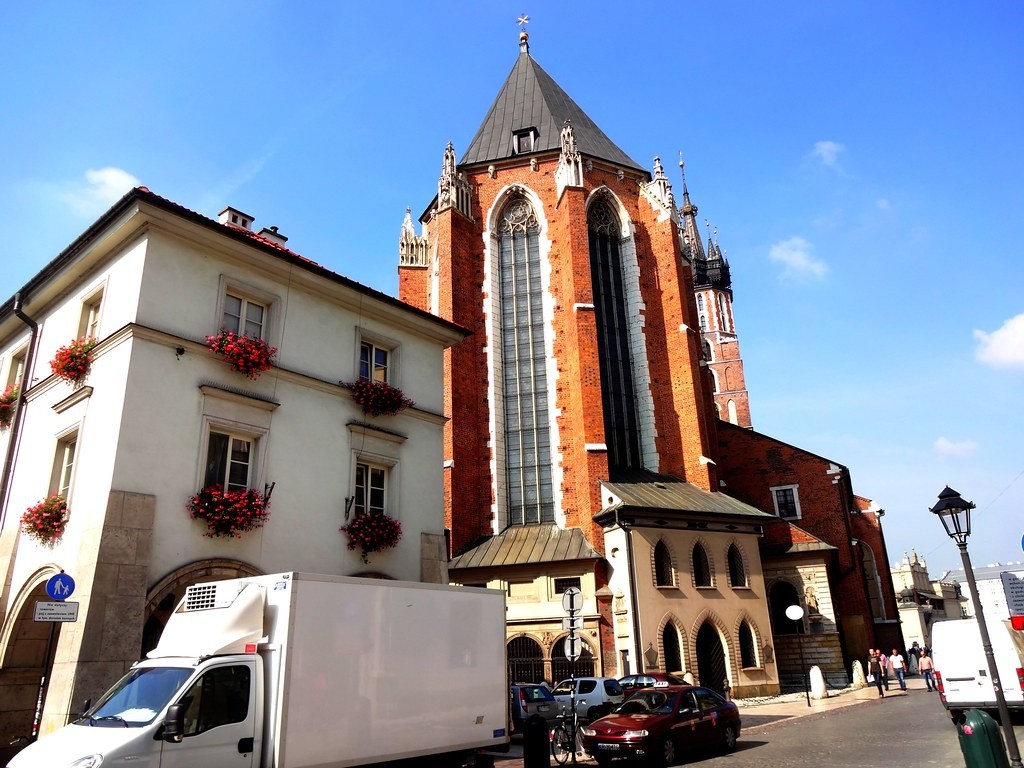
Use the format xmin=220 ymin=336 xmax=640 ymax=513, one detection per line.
xmin=0 ymin=381 xmax=21 ymax=429
xmin=48 ymin=335 xmax=97 ymax=391
xmin=338 ymin=512 xmax=403 ymax=566
xmin=185 ymin=483 xmax=271 ymax=543
xmin=339 ymin=376 xmax=416 ymax=418
xmin=204 ymin=325 xmax=280 ymax=383
xmin=19 ymin=488 xmax=70 ymax=550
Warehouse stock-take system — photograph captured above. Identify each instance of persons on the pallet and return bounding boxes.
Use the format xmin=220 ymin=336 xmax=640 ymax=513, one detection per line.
xmin=889 ymin=649 xmax=907 ymax=690
xmin=910 ymin=642 xmax=938 ymax=692
xmin=867 ymin=649 xmax=885 ymax=699
xmin=875 ymin=649 xmax=889 ymax=691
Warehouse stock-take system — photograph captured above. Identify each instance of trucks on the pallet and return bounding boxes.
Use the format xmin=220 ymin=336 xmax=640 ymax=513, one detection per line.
xmin=4 ymin=570 xmax=509 ymax=768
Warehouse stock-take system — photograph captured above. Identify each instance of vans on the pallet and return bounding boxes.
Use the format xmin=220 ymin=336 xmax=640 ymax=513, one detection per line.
xmin=931 ymin=618 xmax=1024 ymax=724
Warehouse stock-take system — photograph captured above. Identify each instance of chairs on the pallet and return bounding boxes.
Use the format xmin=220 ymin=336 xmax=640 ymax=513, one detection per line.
xmin=183 ymin=686 xmax=202 ymax=734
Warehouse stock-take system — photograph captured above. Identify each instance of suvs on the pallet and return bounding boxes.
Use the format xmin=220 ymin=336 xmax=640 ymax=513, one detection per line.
xmin=510 ymin=684 xmax=561 ymax=736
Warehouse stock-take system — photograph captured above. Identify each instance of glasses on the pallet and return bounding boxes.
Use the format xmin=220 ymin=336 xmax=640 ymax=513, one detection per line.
xmin=921 ymin=654 xmax=925 ymax=656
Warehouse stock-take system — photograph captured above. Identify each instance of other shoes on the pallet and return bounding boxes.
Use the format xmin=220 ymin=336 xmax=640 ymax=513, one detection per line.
xmin=927 ymin=689 xmax=932 ymax=692
xmin=935 ymin=688 xmax=938 ymax=691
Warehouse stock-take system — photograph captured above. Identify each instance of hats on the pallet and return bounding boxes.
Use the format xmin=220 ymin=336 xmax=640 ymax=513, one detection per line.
xmin=912 ymin=642 xmax=918 ymax=645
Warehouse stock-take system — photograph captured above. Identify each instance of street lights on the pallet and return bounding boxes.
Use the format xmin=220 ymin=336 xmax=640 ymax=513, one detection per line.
xmin=929 ymin=486 xmax=1023 ymax=768
xmin=786 ymin=604 xmax=811 ymax=707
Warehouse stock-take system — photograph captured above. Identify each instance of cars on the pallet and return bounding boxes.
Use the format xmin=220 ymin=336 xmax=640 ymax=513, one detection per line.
xmin=618 ymin=672 xmax=693 ymax=700
xmin=552 ymin=677 xmax=625 ymax=721
xmin=582 ymin=682 xmax=744 ymax=764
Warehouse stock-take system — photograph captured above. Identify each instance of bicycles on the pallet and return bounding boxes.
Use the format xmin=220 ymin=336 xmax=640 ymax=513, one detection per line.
xmin=550 ymin=699 xmax=592 ymax=765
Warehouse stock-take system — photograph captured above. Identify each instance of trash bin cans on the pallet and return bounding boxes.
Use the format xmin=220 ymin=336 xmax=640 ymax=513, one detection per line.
xmin=957 ymin=707 xmax=1009 ymax=768
xmin=523 ymin=713 xmax=551 ymax=768
